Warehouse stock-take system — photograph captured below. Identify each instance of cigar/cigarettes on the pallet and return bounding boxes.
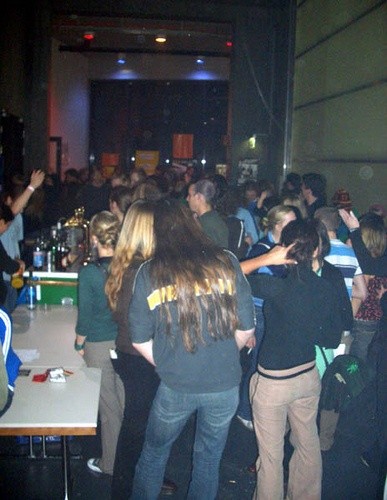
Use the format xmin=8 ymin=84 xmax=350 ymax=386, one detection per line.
xmin=247 ymin=348 xmax=252 ymax=355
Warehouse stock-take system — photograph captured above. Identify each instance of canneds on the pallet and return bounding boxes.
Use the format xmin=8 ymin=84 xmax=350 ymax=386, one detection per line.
xmin=25 ymin=284 xmax=36 ymax=310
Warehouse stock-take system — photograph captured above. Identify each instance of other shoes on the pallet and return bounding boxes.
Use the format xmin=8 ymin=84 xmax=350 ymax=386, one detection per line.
xmin=87 ymin=458 xmax=100 ymax=471
xmin=160 ymin=479 xmax=177 ymax=496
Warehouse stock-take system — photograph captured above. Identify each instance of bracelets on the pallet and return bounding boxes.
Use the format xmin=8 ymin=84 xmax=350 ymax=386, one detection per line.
xmin=27 ymin=185 xmax=35 ymax=192
xmin=75 ymin=341 xmax=84 ymax=351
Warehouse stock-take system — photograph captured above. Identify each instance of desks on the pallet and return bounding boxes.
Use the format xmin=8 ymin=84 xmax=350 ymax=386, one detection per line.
xmin=0 ymin=303 xmax=102 ymax=500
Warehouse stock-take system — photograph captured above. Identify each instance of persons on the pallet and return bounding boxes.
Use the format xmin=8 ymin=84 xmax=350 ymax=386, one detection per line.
xmin=126 ymin=198 xmax=256 ymax=500
xmin=237 ymin=220 xmax=342 ymax=500
xmin=0 ymin=153 xmax=386 ymax=500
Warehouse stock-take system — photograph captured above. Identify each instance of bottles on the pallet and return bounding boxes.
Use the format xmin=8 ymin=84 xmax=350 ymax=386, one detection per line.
xmin=27 ymin=270 xmax=36 ymax=310
xmin=33 ymin=222 xmax=70 ymax=272
xmin=11 ymin=254 xmax=24 ymax=288
